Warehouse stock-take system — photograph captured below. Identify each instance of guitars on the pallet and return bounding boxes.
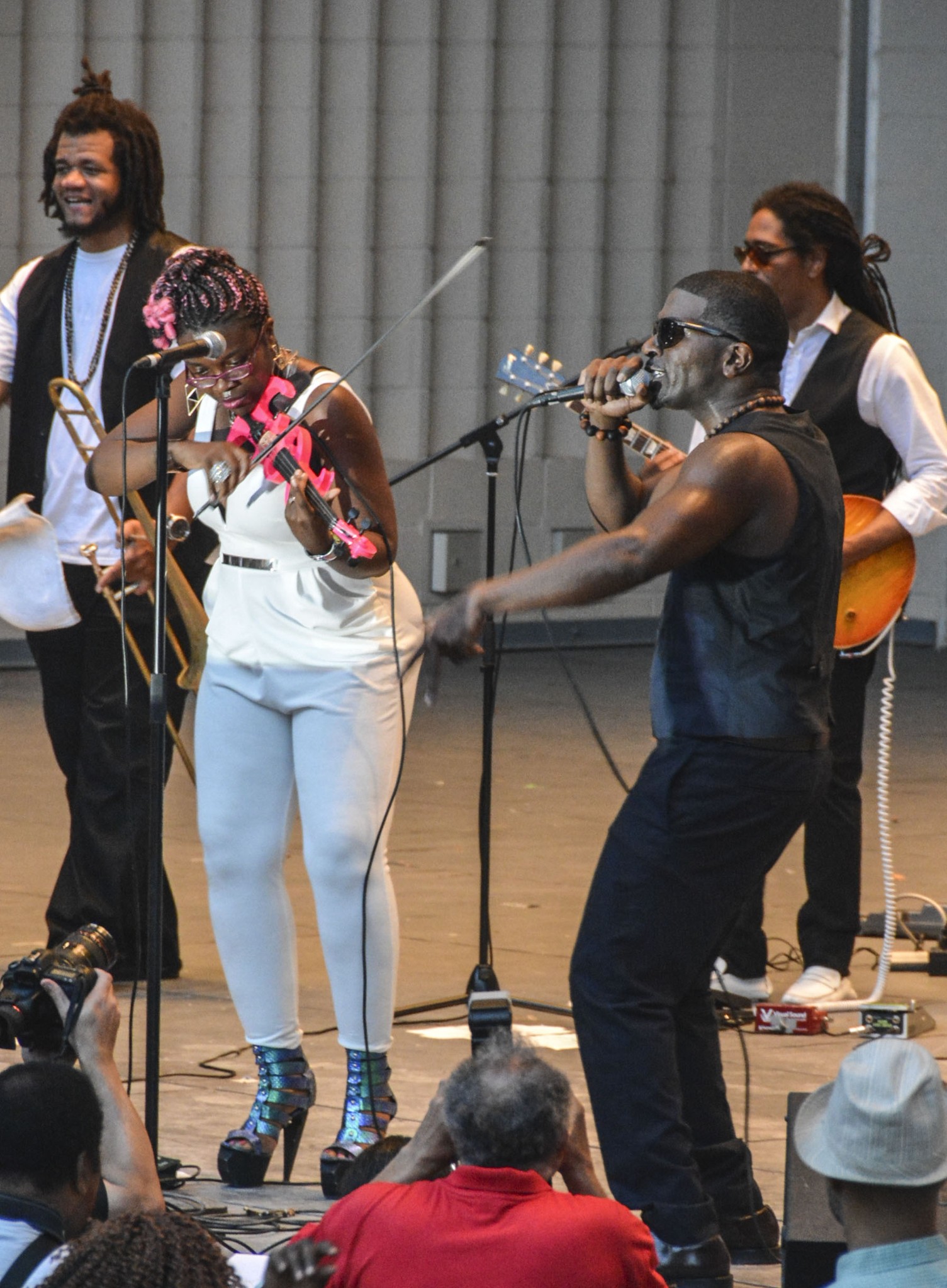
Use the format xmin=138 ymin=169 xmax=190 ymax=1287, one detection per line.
xmin=493 ymin=343 xmax=917 ymax=649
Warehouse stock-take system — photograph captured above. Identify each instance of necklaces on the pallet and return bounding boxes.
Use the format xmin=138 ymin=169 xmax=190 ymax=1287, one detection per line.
xmin=703 ymin=395 xmax=784 ymax=441
xmin=226 ymin=358 xmax=297 ymax=428
xmin=62 ymin=219 xmax=142 ymax=390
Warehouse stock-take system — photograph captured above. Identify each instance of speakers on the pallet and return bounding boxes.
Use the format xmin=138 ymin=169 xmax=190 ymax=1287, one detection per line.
xmin=778 ymin=1089 xmax=947 ymax=1288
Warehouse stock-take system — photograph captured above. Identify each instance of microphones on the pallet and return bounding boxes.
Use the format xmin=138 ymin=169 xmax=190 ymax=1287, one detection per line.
xmin=534 ymin=369 xmax=651 ymax=407
xmin=132 ymin=330 xmax=228 ymax=369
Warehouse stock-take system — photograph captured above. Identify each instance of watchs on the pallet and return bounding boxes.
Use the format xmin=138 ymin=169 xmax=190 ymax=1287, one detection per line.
xmin=164 ymin=512 xmax=191 ymax=542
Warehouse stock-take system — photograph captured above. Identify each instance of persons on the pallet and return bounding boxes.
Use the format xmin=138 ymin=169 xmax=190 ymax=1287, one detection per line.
xmin=688 ymin=180 xmax=947 ymax=1014
xmin=0 ymin=55 xmax=209 ymax=979
xmin=0 ymin=965 xmax=947 ymax=1288
xmin=431 ymin=269 xmax=846 ymax=1288
xmin=86 ymin=245 xmax=423 ymax=1191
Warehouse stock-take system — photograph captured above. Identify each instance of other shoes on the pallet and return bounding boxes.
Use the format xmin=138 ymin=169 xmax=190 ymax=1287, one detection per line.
xmin=709 ymin=957 xmax=773 ymax=1001
xmin=780 ymin=965 xmax=858 ymax=1005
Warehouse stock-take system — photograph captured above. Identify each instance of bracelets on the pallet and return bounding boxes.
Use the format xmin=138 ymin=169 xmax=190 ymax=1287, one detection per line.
xmin=305 ymin=541 xmax=340 ymax=564
xmin=165 ymin=440 xmax=189 ymax=474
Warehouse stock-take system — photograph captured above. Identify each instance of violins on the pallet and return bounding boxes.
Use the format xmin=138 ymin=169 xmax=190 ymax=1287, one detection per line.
xmin=225 ymin=374 xmax=378 ymax=569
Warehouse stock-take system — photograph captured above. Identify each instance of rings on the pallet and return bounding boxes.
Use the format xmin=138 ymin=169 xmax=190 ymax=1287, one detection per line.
xmin=213 ymin=463 xmax=231 ymax=483
xmin=288 ymin=495 xmax=296 ymax=505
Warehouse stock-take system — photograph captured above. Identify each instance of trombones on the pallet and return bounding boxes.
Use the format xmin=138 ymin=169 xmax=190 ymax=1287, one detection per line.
xmin=48 ymin=377 xmax=210 ymax=789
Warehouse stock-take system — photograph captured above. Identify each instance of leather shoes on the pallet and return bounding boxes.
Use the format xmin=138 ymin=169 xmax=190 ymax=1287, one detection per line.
xmin=649 ymin=1231 xmax=733 ymax=1288
xmin=720 ymin=1204 xmax=781 ymax=1265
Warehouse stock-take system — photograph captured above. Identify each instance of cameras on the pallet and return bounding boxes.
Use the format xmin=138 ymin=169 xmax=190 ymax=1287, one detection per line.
xmin=0 ymin=924 xmax=119 ymax=1058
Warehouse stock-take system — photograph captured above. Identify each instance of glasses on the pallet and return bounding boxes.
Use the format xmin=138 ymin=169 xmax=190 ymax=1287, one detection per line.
xmin=733 ymin=243 xmax=811 ymax=266
xmin=653 ymin=317 xmax=743 ymax=350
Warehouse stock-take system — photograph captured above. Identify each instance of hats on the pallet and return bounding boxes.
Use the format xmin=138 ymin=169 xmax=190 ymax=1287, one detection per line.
xmin=794 ymin=1037 xmax=947 ymax=1188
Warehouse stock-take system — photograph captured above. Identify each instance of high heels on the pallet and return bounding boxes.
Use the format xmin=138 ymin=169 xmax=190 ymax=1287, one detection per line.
xmin=217 ymin=1044 xmax=317 ymax=1189
xmin=320 ymin=1048 xmax=398 ymax=1200
